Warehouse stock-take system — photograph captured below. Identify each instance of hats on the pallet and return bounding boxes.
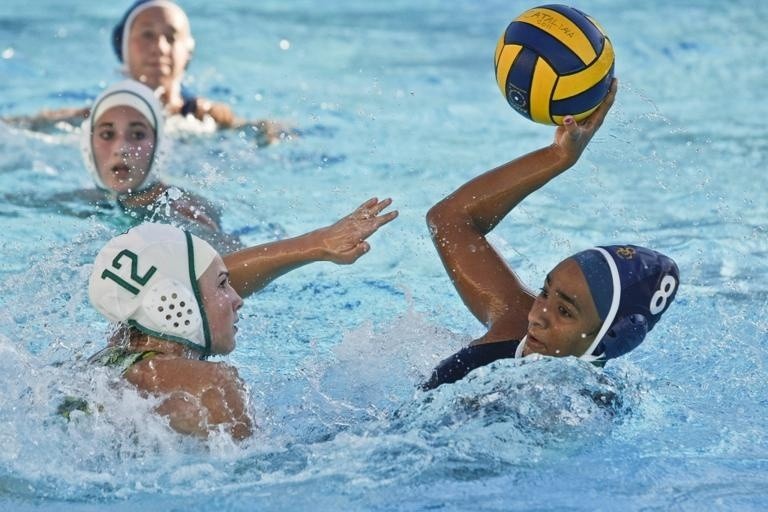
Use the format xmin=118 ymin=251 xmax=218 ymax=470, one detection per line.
xmin=110 ymin=0 xmax=197 ymax=73
xmin=576 ymin=242 xmax=683 ymax=363
xmin=77 ymin=78 xmax=164 ymax=200
xmin=84 ymin=221 xmax=221 ymax=359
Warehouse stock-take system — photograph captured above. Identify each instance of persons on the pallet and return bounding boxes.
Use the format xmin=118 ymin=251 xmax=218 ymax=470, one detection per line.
xmin=0 ymin=81 xmax=238 ymax=250
xmin=45 ymin=197 xmax=399 ymax=439
xmin=238 ymin=77 xmax=680 ymax=477
xmin=0 ymin=0 xmax=291 ymax=150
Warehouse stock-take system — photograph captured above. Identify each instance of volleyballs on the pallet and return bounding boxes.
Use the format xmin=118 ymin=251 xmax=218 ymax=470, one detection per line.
xmin=496 ymin=4 xmax=614 ymax=125
